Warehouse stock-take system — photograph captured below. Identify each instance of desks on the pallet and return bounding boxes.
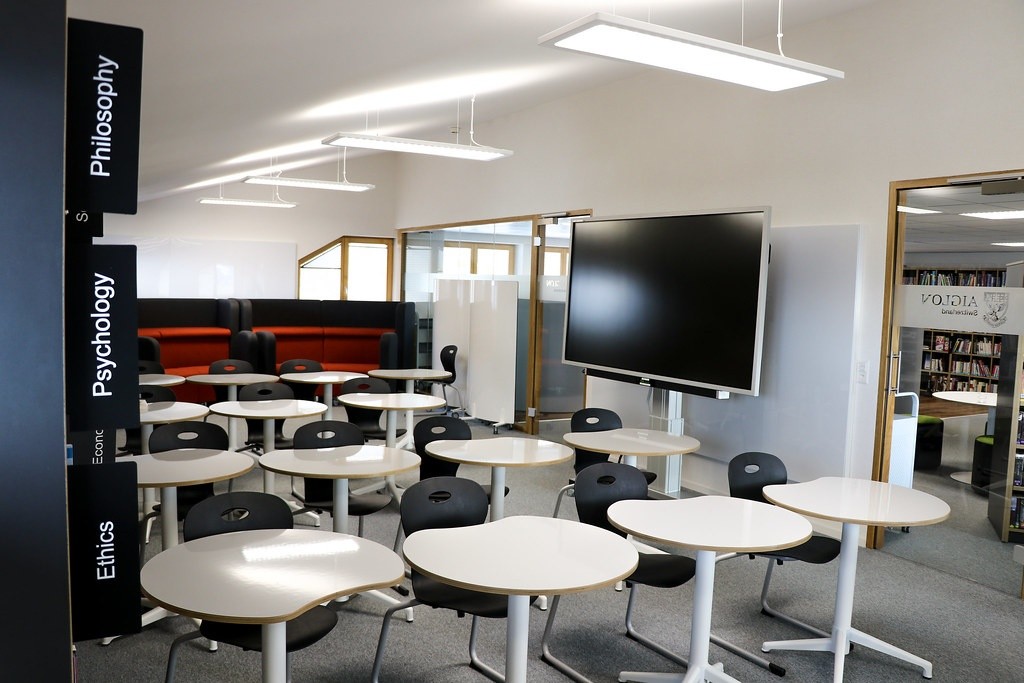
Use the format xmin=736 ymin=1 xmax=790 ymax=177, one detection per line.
xmin=137 ymin=374 xmax=185 ymax=405
xmin=281 ymin=372 xmax=369 ymax=437
xmin=369 ymin=369 xmax=453 ymax=452
xmin=259 ymin=445 xmax=422 ymax=624
xmin=401 ymin=515 xmax=639 ymax=683
xmin=208 ymin=398 xmax=328 ymax=528
xmin=606 ymin=494 xmax=814 ymax=683
xmin=139 ymin=529 xmax=404 ymax=683
xmin=184 ymin=374 xmax=279 ymax=461
xmin=335 ymin=393 xmax=447 ymax=512
xmin=137 ymin=400 xmax=207 ymax=539
xmin=931 ymin=391 xmax=997 ymax=485
xmin=562 ymin=428 xmax=701 ymax=592
xmin=102 ymin=447 xmax=255 ymax=656
xmin=424 ymin=436 xmax=574 ymax=612
xmin=760 ymin=475 xmax=951 ymax=681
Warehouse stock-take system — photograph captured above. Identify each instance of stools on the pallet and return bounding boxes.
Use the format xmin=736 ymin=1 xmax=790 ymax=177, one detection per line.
xmin=900 ymin=413 xmax=944 ymax=473
xmin=971 ymin=435 xmax=994 ymax=490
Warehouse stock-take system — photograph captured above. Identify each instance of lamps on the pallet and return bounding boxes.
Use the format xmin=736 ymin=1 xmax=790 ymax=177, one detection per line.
xmin=321 ymin=93 xmax=515 ymax=162
xmin=195 ymin=155 xmax=299 ymax=209
xmin=239 ymin=145 xmax=376 ymax=192
xmin=536 ymin=0 xmax=846 ymax=93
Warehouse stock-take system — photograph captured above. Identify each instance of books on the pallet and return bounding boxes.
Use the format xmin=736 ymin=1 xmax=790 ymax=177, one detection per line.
xmin=903 ymin=271 xmax=1007 ymax=287
xmin=923 ymin=330 xmax=1001 ymax=393
xmin=1010 ymin=369 xmax=1024 ymax=528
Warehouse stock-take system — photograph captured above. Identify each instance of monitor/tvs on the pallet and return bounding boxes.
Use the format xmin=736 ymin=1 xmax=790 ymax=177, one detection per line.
xmin=561 ymin=205 xmax=772 ymax=397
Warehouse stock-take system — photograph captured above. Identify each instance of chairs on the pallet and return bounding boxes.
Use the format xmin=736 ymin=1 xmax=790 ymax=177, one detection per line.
xmin=340 ymin=378 xmax=407 ymax=445
xmin=279 ymin=360 xmax=323 ymax=442
xmin=141 ymin=421 xmax=230 ymax=586
xmin=710 ymin=452 xmax=841 ymax=677
xmin=540 ymin=464 xmax=689 ymax=683
xmin=369 ymin=476 xmax=505 ymax=683
xmin=163 ymin=490 xmax=338 ymax=683
xmin=137 ymin=336 xmax=166 ymax=374
xmin=229 ymin=382 xmax=324 ymax=515
xmin=137 ymin=385 xmax=176 ymax=431
xmin=404 ymin=345 xmax=466 ymax=419
xmin=552 ymin=407 xmax=657 ymax=520
xmin=204 ymin=359 xmax=252 ymax=422
xmin=392 ymin=417 xmax=509 ymax=580
xmin=291 ymin=419 xmax=410 ymax=597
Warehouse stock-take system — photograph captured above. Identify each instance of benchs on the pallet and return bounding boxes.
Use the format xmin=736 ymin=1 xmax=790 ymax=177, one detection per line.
xmin=137 ymin=297 xmax=261 ymax=413
xmin=229 ymin=298 xmax=415 ymax=406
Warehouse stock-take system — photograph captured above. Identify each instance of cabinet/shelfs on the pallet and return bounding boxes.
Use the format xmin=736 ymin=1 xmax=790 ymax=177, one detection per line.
xmin=902 ymin=266 xmax=1024 ymax=543
